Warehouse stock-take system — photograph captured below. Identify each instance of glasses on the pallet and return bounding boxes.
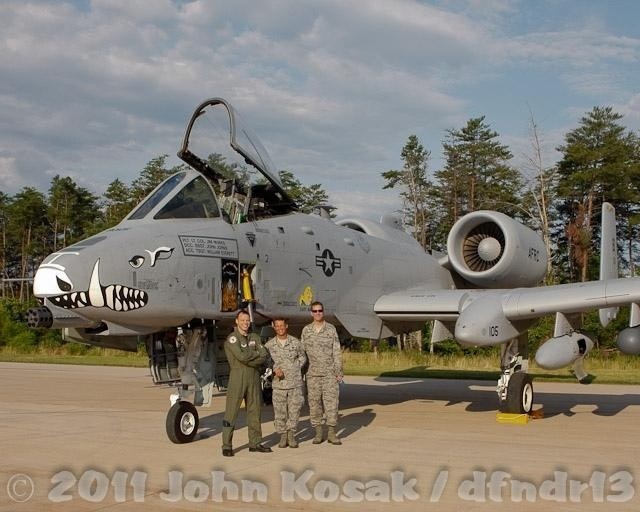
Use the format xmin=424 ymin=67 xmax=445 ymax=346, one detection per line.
xmin=312 ymin=309 xmax=323 ymax=313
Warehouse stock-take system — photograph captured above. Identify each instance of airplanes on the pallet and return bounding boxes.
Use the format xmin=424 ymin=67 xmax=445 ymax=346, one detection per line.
xmin=25 ymin=99 xmax=639 ymax=443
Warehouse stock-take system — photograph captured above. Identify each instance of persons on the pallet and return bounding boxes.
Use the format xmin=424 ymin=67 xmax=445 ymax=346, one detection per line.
xmin=221 ymin=309 xmax=273 ymax=456
xmin=300 ymin=301 xmax=345 ymax=445
xmin=264 ymin=317 xmax=308 ymax=448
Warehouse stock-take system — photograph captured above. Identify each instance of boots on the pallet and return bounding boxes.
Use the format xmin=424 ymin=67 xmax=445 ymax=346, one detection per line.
xmin=312 ymin=425 xmax=324 ymax=444
xmin=279 ymin=432 xmax=288 ymax=448
xmin=287 ymin=429 xmax=298 ymax=448
xmin=327 ymin=425 xmax=341 ymax=445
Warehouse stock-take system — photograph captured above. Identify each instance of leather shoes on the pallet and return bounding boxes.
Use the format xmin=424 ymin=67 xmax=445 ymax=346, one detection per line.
xmin=249 ymin=445 xmax=271 ymax=452
xmin=223 ymin=448 xmax=232 ymax=456
